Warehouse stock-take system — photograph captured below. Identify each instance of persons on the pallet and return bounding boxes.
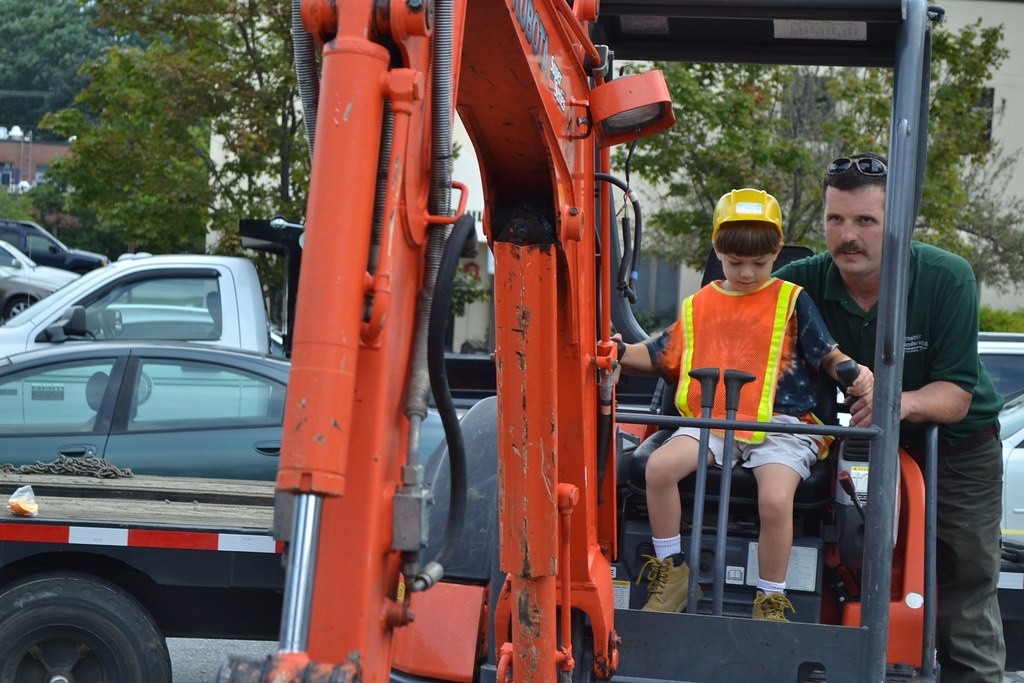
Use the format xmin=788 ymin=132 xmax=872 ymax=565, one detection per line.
xmin=599 ymin=147 xmax=1010 ymax=683
xmin=594 ymin=185 xmax=877 ymax=624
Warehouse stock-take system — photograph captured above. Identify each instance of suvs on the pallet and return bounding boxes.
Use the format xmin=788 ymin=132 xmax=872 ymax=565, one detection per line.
xmin=1 ymin=219 xmax=108 ymax=320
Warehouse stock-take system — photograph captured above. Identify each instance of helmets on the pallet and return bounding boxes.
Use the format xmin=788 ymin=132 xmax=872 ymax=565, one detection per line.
xmin=710 ymin=183 xmax=784 ymax=244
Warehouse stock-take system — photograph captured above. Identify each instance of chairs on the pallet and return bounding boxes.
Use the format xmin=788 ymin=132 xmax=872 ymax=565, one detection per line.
xmin=623 ymin=237 xmax=844 ymax=525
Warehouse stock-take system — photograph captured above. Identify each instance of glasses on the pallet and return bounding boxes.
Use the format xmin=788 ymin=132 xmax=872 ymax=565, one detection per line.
xmin=826 ymin=158 xmax=892 ymax=180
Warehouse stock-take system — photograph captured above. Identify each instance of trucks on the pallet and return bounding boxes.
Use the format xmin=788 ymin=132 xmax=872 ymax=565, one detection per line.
xmin=0 ymin=217 xmax=306 ymax=358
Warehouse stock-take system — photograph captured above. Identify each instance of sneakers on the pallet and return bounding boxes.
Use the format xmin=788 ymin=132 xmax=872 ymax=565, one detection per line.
xmin=635 ymin=552 xmax=703 ymax=616
xmin=751 ymin=591 xmax=794 ymax=630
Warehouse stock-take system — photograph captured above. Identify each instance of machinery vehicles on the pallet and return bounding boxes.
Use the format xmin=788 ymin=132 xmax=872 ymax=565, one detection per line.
xmin=227 ymin=2 xmax=946 ymax=683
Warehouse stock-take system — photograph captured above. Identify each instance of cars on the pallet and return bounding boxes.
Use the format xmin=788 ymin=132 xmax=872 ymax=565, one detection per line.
xmin=0 ymin=341 xmax=468 ymax=493
xmin=824 ymin=325 xmax=1023 ymax=576
